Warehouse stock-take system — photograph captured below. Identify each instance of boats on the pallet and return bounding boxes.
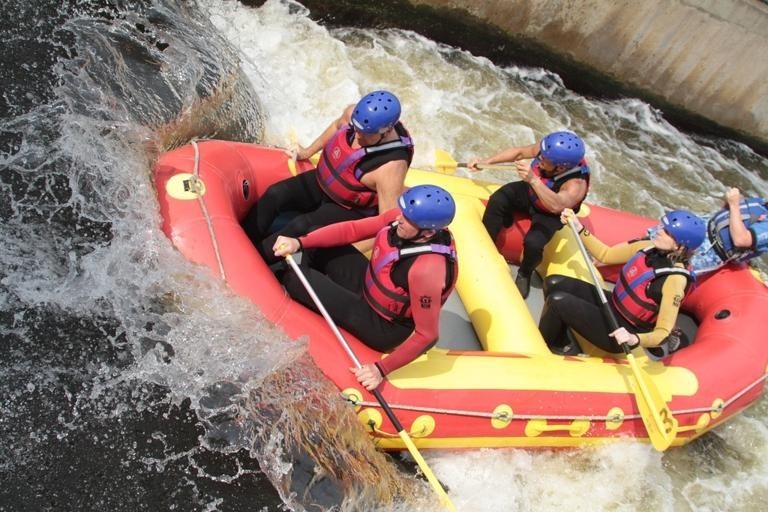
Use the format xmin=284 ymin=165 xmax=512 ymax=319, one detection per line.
xmin=148 ymin=137 xmax=768 ymax=453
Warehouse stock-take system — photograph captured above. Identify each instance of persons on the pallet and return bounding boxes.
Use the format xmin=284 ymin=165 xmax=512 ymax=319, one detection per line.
xmin=268 ymin=184 xmax=460 ymax=392
xmin=587 ymin=186 xmax=767 ymax=278
xmin=241 ymin=90 xmax=414 ymax=264
xmin=536 ymin=208 xmax=707 ymax=354
xmin=468 ymin=130 xmax=589 ymax=300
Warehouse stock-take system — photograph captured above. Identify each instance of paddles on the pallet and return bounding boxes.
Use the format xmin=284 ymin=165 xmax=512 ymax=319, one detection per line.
xmin=426 ymin=148 xmax=519 ymax=175
xmin=563 ymin=214 xmax=678 ymax=453
xmin=286 ymin=253 xmax=455 ymax=511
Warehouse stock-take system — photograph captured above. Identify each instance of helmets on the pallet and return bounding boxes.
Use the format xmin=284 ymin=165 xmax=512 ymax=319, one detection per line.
xmin=661 ymin=209 xmax=706 ymax=250
xmin=397 ymin=185 xmax=456 ymax=230
xmin=540 ymin=131 xmax=585 ymax=170
xmin=349 ymin=91 xmax=402 ymax=135
